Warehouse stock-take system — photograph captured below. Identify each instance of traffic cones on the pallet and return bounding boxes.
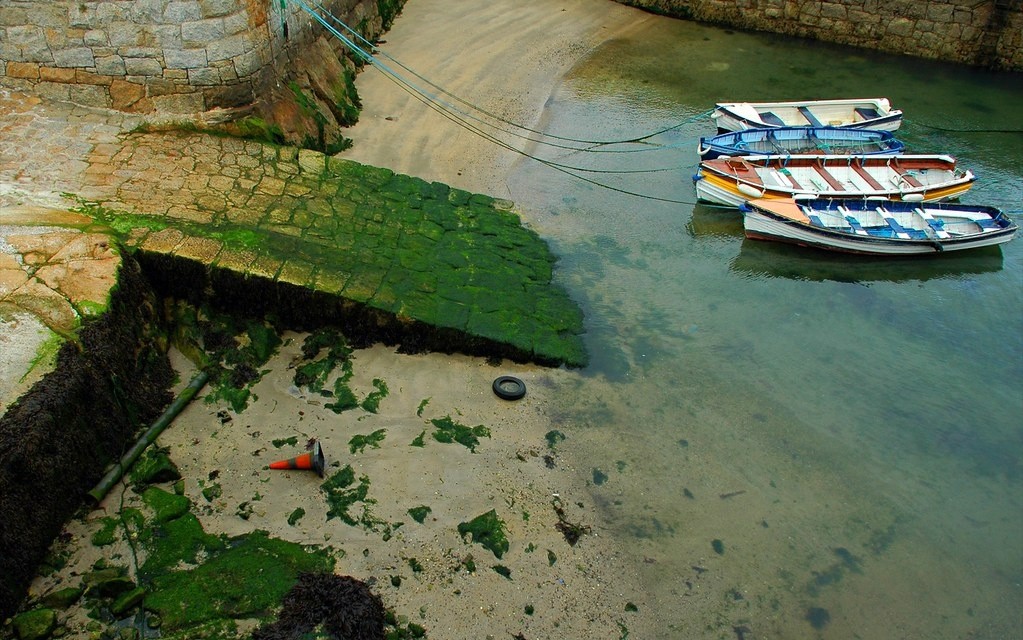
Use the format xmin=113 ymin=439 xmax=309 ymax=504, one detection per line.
xmin=269 ymin=440 xmax=325 ymax=478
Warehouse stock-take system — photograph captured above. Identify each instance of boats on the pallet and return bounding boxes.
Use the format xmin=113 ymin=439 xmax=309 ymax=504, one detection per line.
xmin=692 ymin=97 xmax=1019 ymax=259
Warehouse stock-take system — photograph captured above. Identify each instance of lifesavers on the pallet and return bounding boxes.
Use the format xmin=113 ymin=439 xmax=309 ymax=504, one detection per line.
xmin=491 ymin=375 xmax=528 ymax=400
